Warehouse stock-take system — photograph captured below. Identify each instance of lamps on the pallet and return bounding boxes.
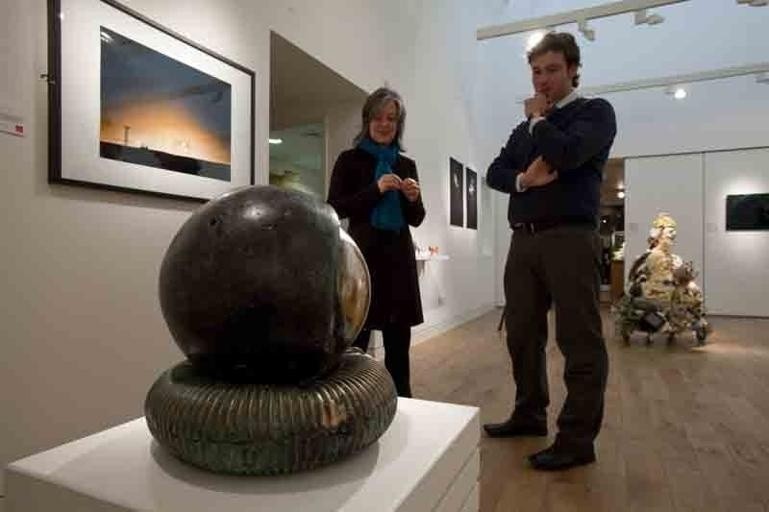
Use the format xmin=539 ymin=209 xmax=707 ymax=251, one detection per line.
xmin=476 ymin=1 xmax=768 ymax=47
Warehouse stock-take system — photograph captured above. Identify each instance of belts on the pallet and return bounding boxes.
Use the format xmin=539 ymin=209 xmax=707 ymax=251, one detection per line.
xmin=514 ymin=217 xmax=591 ymax=234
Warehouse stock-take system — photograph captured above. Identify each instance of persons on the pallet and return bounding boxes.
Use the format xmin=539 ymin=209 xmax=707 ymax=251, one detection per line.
xmin=322 ymin=86 xmax=426 ymax=399
xmin=480 ymin=30 xmax=620 ymax=474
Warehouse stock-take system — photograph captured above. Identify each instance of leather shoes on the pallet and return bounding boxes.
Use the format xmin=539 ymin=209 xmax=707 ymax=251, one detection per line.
xmin=483 ymin=421 xmax=547 ymax=437
xmin=528 ymin=444 xmax=595 ymax=470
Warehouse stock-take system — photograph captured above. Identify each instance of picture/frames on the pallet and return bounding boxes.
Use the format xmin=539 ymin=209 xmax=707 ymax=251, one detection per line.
xmin=447 ymin=155 xmax=465 ymax=228
xmin=464 ymin=167 xmax=478 ymax=229
xmin=46 ymin=1 xmax=259 ymax=206
